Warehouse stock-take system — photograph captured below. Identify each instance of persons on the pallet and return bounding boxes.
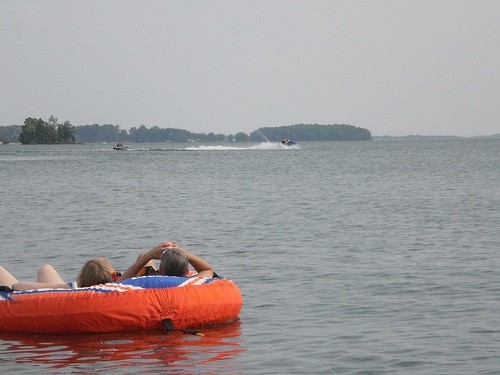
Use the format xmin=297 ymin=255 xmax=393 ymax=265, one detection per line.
xmin=98 ymin=240 xmax=214 ymax=283
xmin=0 ymin=257 xmax=116 ymax=291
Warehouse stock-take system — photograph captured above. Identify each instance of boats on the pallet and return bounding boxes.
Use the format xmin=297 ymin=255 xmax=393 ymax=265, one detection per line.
xmin=113 ymin=146 xmax=131 ymax=150
xmin=282 ymin=137 xmax=298 ymax=146
xmin=0 ymin=273 xmax=244 ymax=333
